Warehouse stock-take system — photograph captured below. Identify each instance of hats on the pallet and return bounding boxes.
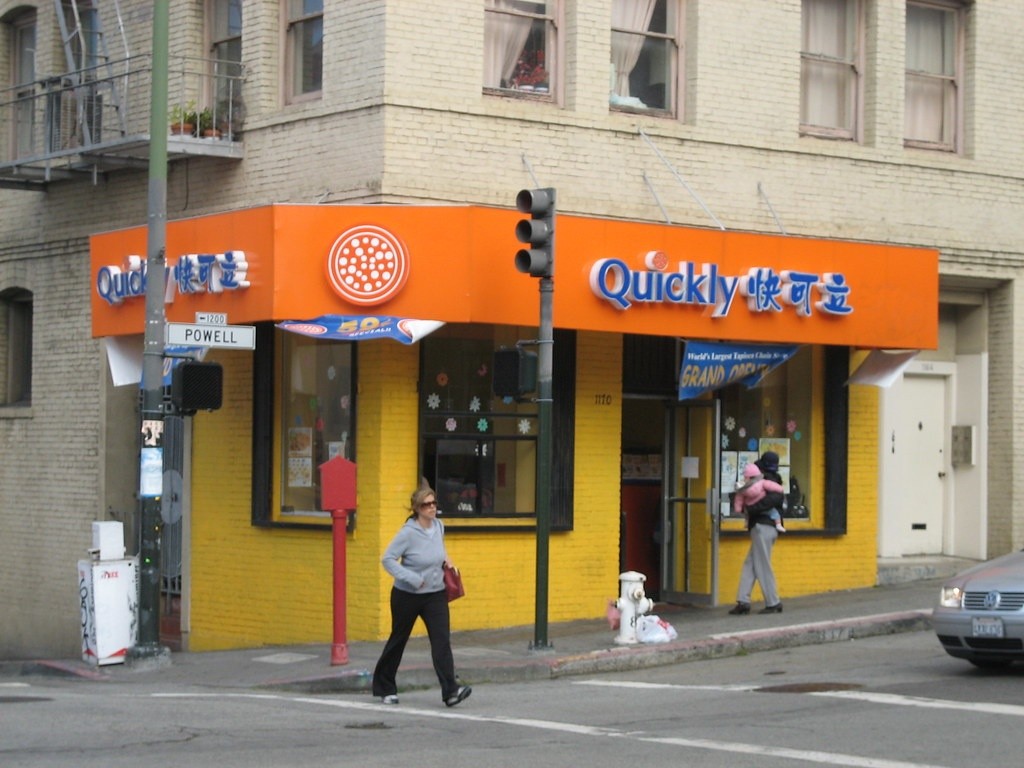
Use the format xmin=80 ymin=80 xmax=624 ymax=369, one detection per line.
xmin=755 ymin=452 xmax=778 ymax=471
xmin=740 ymin=463 xmax=761 ymax=476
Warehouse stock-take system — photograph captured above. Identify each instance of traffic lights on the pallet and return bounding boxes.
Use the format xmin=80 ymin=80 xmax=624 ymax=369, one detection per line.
xmin=172 ymin=362 xmax=223 ymax=410
xmin=515 ymin=189 xmax=552 ymax=278
xmin=493 ymin=349 xmax=538 ymax=396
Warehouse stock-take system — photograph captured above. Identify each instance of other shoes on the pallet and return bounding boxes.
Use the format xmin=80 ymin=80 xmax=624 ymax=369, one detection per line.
xmin=760 ymin=603 xmax=783 ymax=614
xmin=383 ymin=695 xmax=398 ymax=704
xmin=729 ymin=605 xmax=750 ymax=614
xmin=446 ymin=685 xmax=471 ymax=707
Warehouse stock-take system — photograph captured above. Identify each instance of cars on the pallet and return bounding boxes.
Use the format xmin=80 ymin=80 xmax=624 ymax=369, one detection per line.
xmin=933 ymin=544 xmax=1024 ymax=677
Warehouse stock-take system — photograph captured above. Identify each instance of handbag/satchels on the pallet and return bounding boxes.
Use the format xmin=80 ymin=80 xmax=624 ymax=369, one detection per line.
xmin=443 ymin=565 xmax=465 ymax=602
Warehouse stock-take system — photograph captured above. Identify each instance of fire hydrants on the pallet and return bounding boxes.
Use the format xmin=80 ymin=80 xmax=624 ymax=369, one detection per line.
xmin=613 ymin=570 xmax=653 ymax=646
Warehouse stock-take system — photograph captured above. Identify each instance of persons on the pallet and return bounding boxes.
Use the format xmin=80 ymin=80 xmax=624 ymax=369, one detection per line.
xmin=728 ymin=452 xmax=783 ymax=615
xmin=371 ymin=476 xmax=472 ymax=708
xmin=734 ymin=463 xmax=787 ymax=533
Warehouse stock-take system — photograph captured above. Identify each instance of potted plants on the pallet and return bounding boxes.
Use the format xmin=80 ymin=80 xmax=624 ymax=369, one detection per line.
xmin=171 ymin=100 xmax=235 ymax=139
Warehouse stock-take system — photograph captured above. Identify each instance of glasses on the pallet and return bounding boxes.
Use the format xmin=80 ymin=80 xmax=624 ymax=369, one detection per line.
xmin=422 ymin=501 xmax=438 ymax=508
